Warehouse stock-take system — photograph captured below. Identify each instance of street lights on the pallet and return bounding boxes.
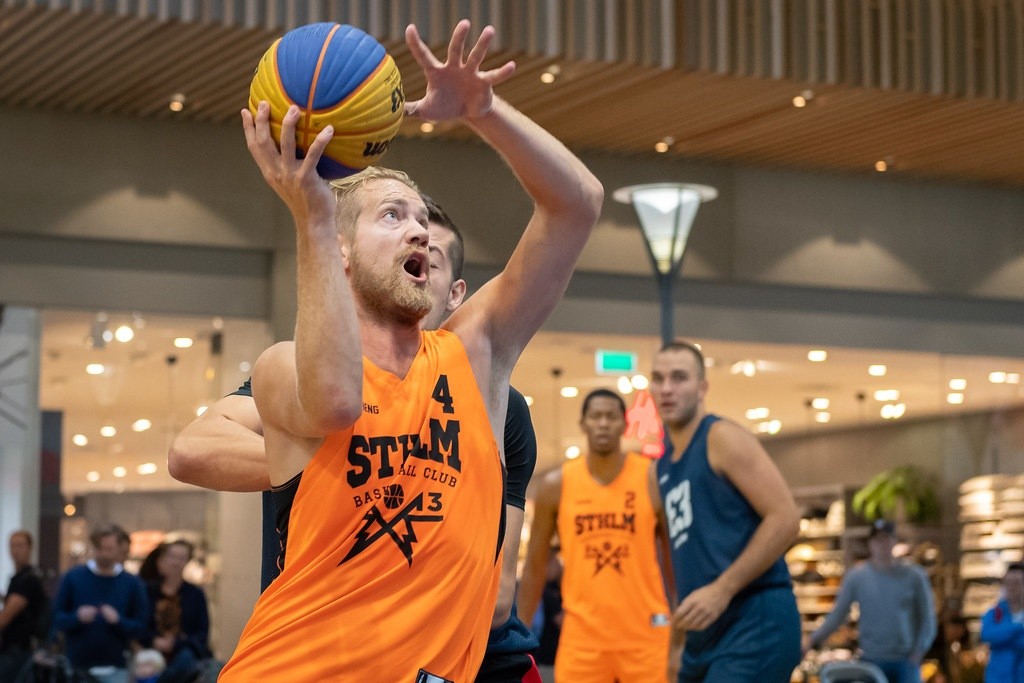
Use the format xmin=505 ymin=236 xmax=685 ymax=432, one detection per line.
xmin=613 ymin=183 xmax=720 ymax=348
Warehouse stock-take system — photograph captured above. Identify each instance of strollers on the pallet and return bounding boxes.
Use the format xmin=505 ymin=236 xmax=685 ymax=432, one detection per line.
xmin=809 ymin=652 xmax=891 ymax=683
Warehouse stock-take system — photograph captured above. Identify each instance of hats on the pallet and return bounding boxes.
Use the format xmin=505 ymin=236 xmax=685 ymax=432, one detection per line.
xmin=871 ymin=519 xmax=894 ymax=536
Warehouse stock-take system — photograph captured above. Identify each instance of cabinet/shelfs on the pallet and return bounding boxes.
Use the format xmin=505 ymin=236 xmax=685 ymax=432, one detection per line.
xmin=787 ymin=483 xmax=961 ymax=653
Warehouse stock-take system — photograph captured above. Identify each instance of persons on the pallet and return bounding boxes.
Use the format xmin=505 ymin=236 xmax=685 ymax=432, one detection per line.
xmin=981 ymin=563 xmax=1024 ymax=683
xmin=168 ymin=19 xmax=603 ymax=683
xmin=801 ymin=516 xmax=971 ymax=683
xmin=516 ymin=342 xmax=804 ymax=683
xmin=0 ymin=526 xmax=224 ymax=683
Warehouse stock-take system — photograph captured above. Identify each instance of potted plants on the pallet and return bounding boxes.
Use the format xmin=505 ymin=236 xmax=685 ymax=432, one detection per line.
xmin=852 ymin=462 xmax=944 ymax=522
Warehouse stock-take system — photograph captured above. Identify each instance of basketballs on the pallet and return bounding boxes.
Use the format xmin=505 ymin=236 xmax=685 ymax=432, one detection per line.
xmin=245 ymin=17 xmax=408 ymax=183
